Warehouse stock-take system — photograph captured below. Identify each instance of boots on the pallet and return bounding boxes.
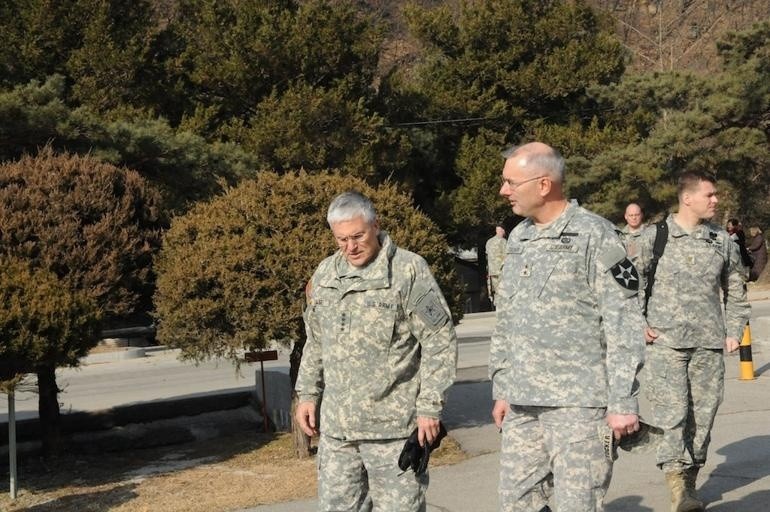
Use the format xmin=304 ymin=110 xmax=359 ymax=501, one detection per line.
xmin=667 ymin=467 xmax=706 ymax=512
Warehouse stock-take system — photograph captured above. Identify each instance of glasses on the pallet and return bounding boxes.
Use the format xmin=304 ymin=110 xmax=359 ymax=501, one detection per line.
xmin=499 ymin=174 xmax=545 ymax=189
xmin=336 ymin=233 xmax=367 ymax=248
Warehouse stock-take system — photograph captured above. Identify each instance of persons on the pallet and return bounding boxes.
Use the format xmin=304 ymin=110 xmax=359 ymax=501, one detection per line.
xmin=726 ymin=218 xmax=755 ymax=269
xmin=293 ymin=189 xmax=461 ymax=512
xmin=486 ymin=140 xmax=647 ymax=512
xmin=746 ymin=226 xmax=768 ymax=284
xmin=628 ymin=170 xmax=753 ymax=512
xmin=485 ymin=224 xmax=508 ymax=312
xmin=622 ymin=202 xmax=647 ymax=249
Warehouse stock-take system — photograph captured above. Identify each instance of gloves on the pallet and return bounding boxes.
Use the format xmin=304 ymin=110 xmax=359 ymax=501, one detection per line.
xmin=399 ymin=421 xmax=447 ymax=476
